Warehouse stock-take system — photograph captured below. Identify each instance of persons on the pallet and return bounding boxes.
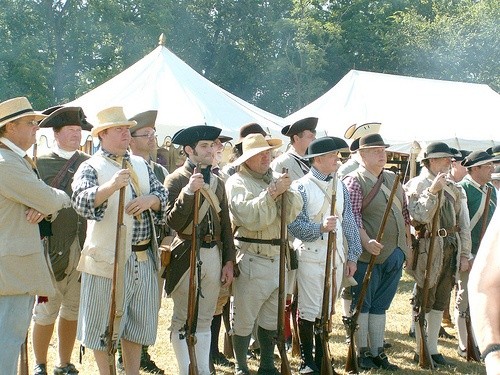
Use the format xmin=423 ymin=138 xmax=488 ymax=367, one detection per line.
xmin=343 ymin=133 xmax=399 ymax=369
xmin=35 ymin=105 xmax=500 ymax=375
xmin=0 ymin=97 xmax=71 ymax=375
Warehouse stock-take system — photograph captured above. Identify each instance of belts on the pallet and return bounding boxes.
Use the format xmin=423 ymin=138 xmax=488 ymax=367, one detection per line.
xmin=236 ymin=236 xmax=281 ymax=245
xmin=415 ymin=226 xmax=461 ymax=237
xmin=131 ymin=242 xmax=151 ymax=252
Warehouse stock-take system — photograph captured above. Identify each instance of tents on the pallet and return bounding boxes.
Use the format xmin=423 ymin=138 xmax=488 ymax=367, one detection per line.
xmin=283 ymin=70 xmax=500 ymax=156
xmin=24 ymin=32 xmax=301 ymax=157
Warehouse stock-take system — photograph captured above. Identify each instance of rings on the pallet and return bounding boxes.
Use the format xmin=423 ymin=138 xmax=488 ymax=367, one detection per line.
xmin=37 ymin=213 xmax=42 ymax=216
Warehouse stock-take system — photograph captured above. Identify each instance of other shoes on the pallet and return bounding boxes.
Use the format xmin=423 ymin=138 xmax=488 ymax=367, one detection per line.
xmin=34 ymin=363 xmax=47 ymax=375
xmin=383 ymin=339 xmax=392 ymax=348
xmin=409 ymin=324 xmax=456 ymax=340
xmin=414 ymin=352 xmax=454 ymax=368
xmin=117 ymin=356 xmax=165 ymax=374
xmin=457 ymin=347 xmax=481 ymax=359
xmin=54 ymin=364 xmax=79 ymax=375
xmin=346 ymin=333 xmax=351 ymax=344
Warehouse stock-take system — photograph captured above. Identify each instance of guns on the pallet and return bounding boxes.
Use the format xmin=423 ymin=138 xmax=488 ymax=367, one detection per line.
xmin=178 ymin=162 xmax=202 ymax=375
xmin=464 ymin=186 xmax=492 ymax=362
xmin=100 ymin=156 xmax=130 ymax=375
xmin=19 ymin=144 xmax=38 ymax=375
xmin=291 ymin=279 xmax=302 ymax=359
xmin=410 ymin=189 xmax=444 ymax=370
xmin=340 ymin=172 xmax=402 ymax=375
xmin=314 ymin=172 xmax=339 ymax=375
xmin=274 ymin=167 xmax=292 ymax=375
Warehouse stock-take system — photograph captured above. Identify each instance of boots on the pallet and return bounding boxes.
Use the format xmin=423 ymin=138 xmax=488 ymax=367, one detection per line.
xmin=211 ymin=314 xmax=234 ymax=368
xmin=298 ymin=317 xmax=337 ymax=375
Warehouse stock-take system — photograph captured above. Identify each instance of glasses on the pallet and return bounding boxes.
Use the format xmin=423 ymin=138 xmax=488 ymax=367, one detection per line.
xmin=134 ymin=132 xmax=158 ymax=138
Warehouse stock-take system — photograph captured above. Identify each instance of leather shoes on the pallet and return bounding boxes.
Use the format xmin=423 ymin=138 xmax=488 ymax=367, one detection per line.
xmin=357 ymin=346 xmax=398 ymax=370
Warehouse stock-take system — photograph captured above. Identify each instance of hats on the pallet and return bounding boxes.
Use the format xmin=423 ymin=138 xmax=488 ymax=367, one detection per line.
xmin=38 ymin=106 xmax=94 ymax=132
xmin=235 ymin=122 xmax=271 ymax=145
xmin=171 ymin=125 xmax=232 ymax=145
xmin=128 ymin=111 xmax=157 ymax=134
xmin=90 ymin=106 xmax=138 ymax=137
xmin=304 ymin=123 xmax=390 ymax=159
xmin=230 ymin=134 xmax=283 ymax=166
xmin=421 ymin=141 xmax=500 ymax=168
xmin=0 ymin=96 xmax=49 ymax=129
xmin=281 ymin=117 xmax=319 ymax=137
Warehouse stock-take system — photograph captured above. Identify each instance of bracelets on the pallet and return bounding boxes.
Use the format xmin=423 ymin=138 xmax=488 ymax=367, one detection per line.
xmin=480 ymin=343 xmax=500 ymax=363
